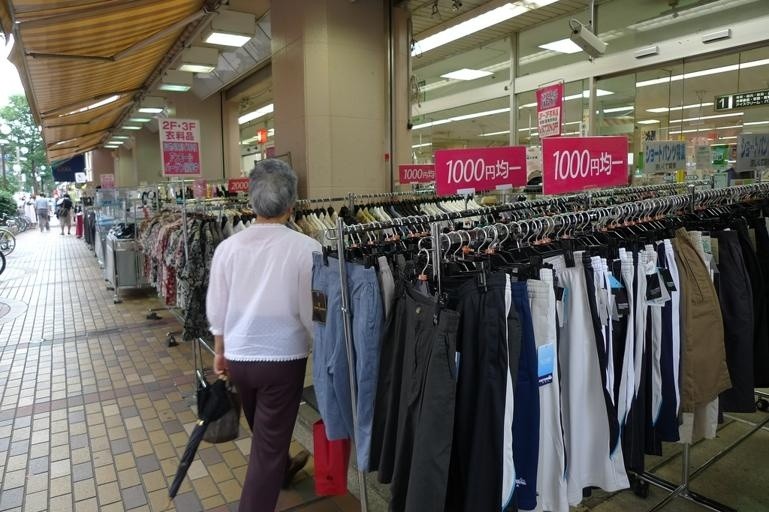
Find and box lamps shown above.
[101,8,256,148]
[450,0,462,13]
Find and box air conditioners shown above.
[702,29,729,42]
[633,46,658,59]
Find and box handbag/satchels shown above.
[203,392,240,442]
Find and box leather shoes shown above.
[282,451,308,488]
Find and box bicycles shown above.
[0,198,32,274]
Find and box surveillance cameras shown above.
[570,23,608,59]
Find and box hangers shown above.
[327,182,695,315]
[183,182,527,316]
[413,182,768,313]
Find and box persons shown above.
[20,186,100,238]
[205,160,325,512]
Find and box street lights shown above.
[40,171,46,192]
[0,139,9,191]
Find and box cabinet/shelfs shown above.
[100,227,152,305]
[92,185,157,269]
